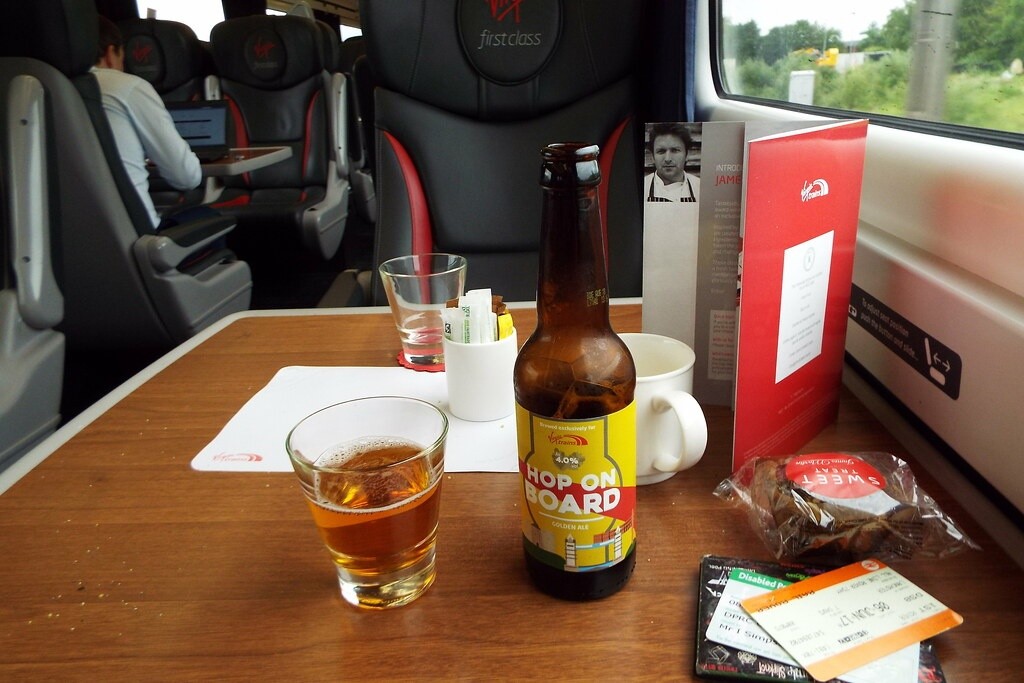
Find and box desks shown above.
[146,145,294,206]
[0,298,1024,683]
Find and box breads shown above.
[749,455,900,564]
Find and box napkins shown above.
[189,364,521,473]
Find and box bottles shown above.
[512,143,640,603]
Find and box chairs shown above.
[210,14,377,265]
[352,0,700,307]
[118,18,204,104]
[0,71,69,477]
[0,57,255,346]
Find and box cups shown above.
[286,396,449,612]
[442,327,518,421]
[617,332,708,487]
[379,253,467,367]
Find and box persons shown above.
[88,14,224,268]
[644,124,699,202]
